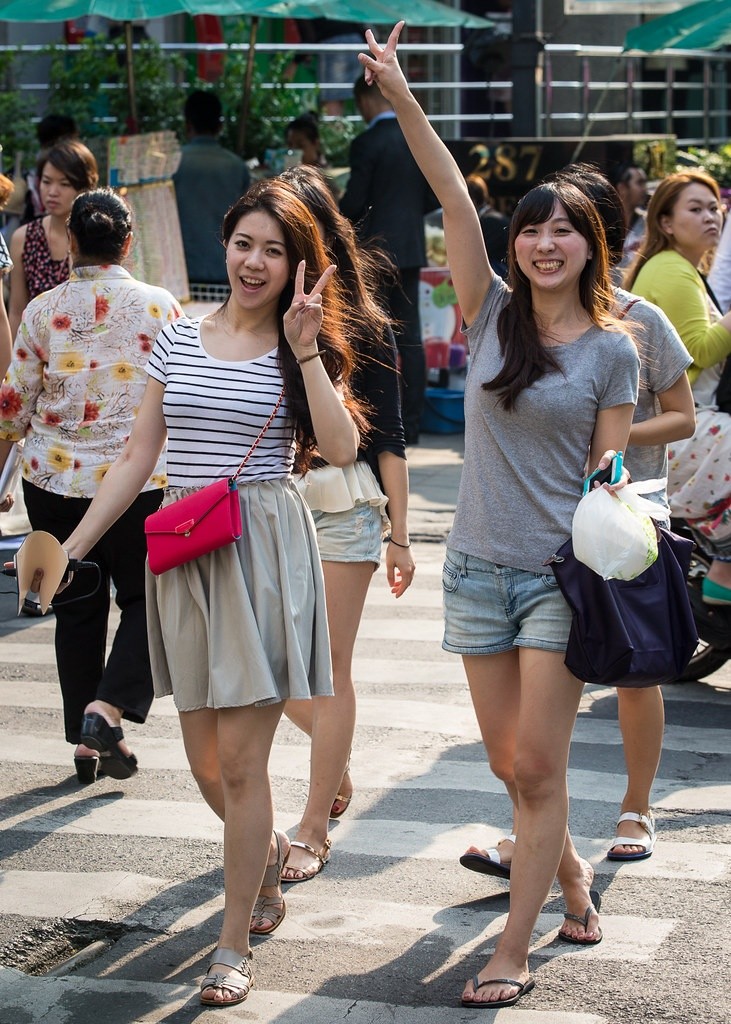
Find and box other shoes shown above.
[698,576,730,606]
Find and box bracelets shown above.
[296,350,326,364]
[389,537,411,548]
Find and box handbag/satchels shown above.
[0,442,27,508]
[143,475,245,576]
[568,475,673,583]
[544,512,701,686]
[715,351,731,415]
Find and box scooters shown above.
[662,518,731,683]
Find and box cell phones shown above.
[584,451,624,496]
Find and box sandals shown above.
[605,813,657,861]
[280,839,332,885]
[325,751,354,815]
[243,830,288,938]
[196,949,256,1007]
[459,834,517,879]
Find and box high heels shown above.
[82,710,139,780]
[74,753,108,783]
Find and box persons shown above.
[174,91,251,304]
[459,172,696,877]
[285,111,327,167]
[339,74,440,445]
[3,187,362,1006]
[272,167,416,881]
[358,21,639,1009]
[611,165,731,605]
[0,139,186,780]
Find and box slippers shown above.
[459,970,537,1008]
[556,889,604,946]
